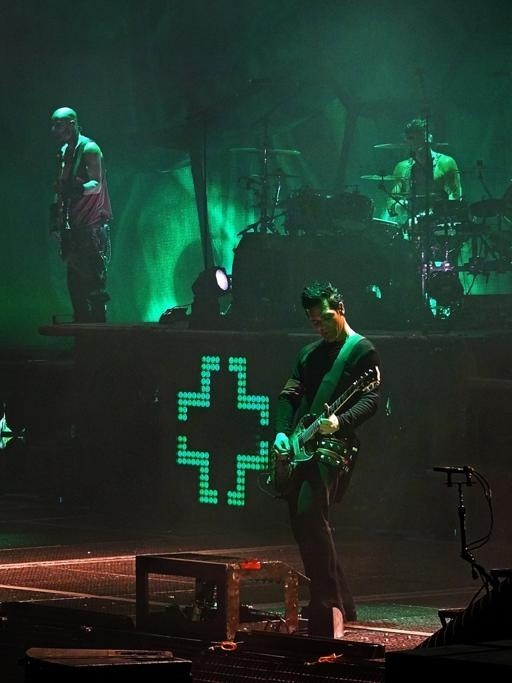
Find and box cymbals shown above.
[229,144,299,156]
[375,140,448,149]
[360,174,402,180]
[253,168,303,180]
[471,198,507,218]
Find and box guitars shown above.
[57,142,85,262]
[272,366,381,489]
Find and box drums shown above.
[332,192,375,233]
[288,187,334,236]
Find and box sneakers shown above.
[301,605,357,622]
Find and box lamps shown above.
[189,267,231,330]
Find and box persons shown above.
[268,275,382,623]
[45,103,115,324]
[376,118,463,223]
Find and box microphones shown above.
[431,465,475,474]
